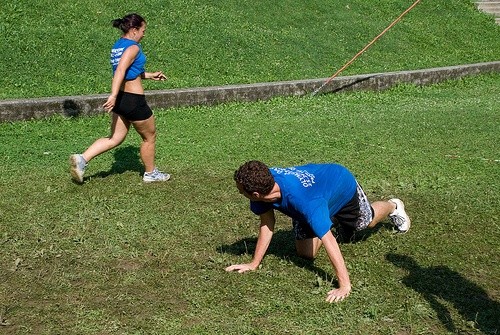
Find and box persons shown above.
[69,12,171,183]
[224,159,411,303]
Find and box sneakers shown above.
[69,154,88,184]
[143,168,170,182]
[387,198,411,232]
